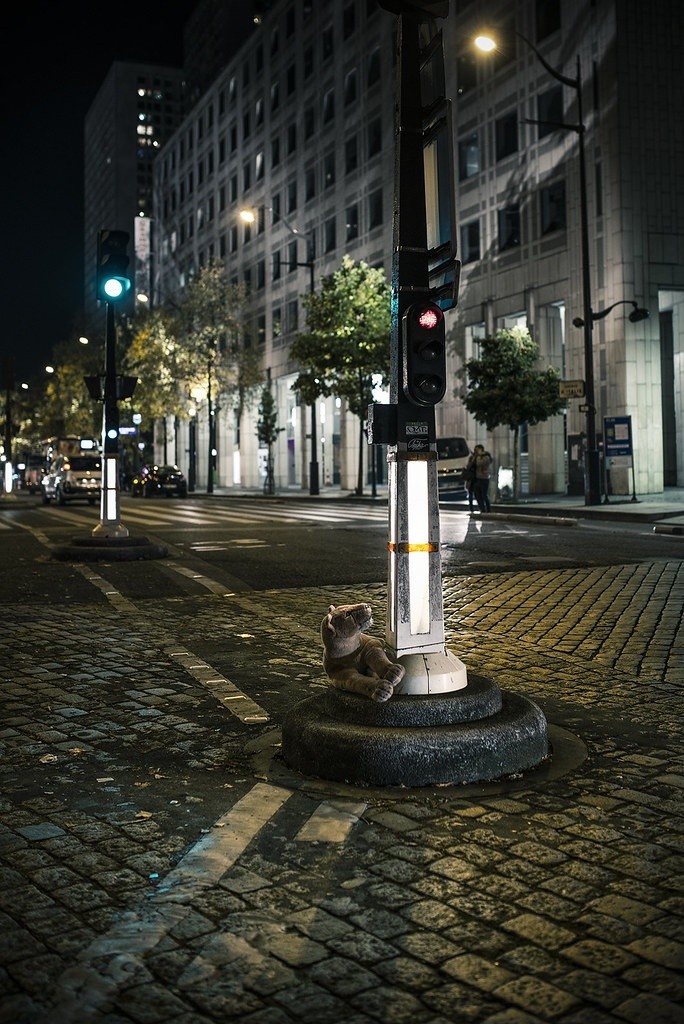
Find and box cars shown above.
[118,465,139,492]
[131,465,188,499]
[0,448,54,495]
[435,435,474,496]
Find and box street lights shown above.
[471,23,651,505]
[241,202,322,496]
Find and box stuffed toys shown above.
[319,602,406,703]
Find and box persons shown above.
[466,452,483,513]
[474,444,494,512]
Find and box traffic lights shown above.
[96,227,132,306]
[399,300,447,410]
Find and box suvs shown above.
[40,453,102,506]
